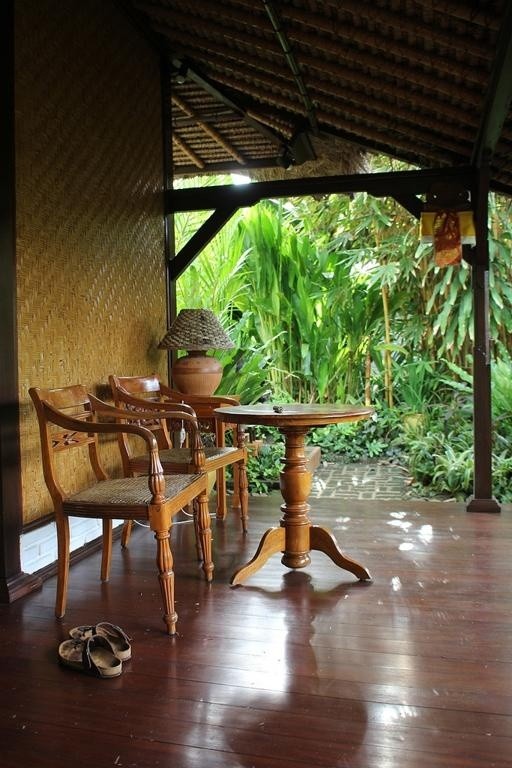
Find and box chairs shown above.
[28,374,249,636]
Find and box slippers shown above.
[59,634,122,678]
[70,622,132,662]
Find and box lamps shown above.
[156,308,236,397]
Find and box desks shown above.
[214,401,377,589]
